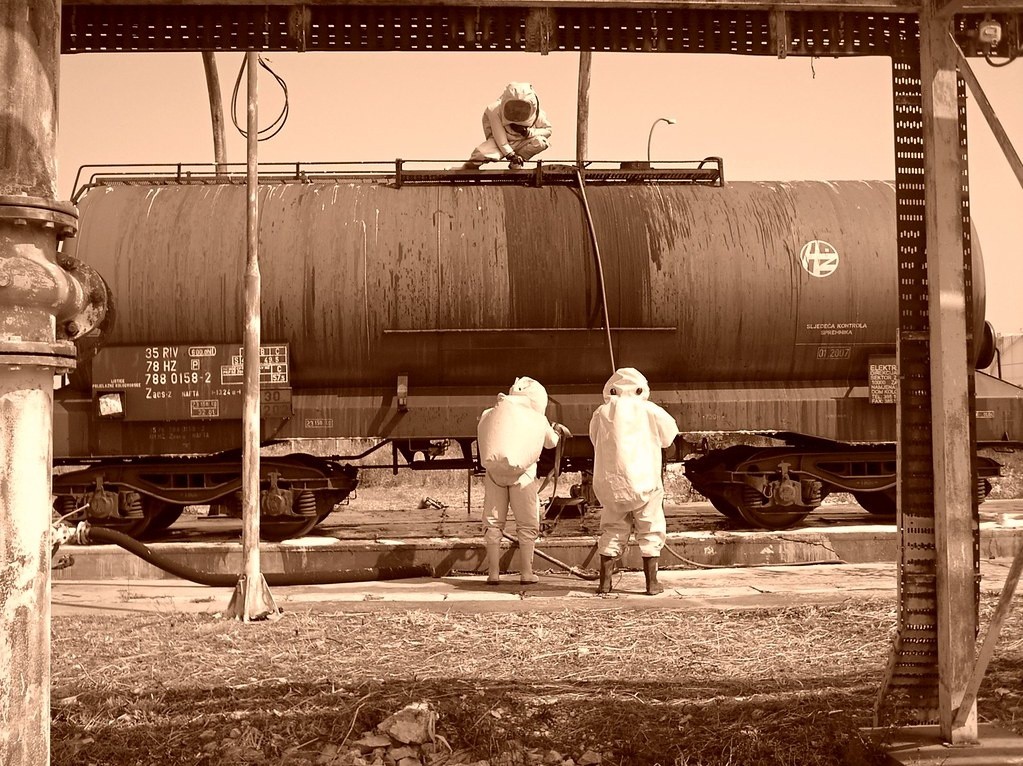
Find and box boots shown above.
[486,543,501,585]
[642,556,665,595]
[596,553,615,593]
[519,541,539,585]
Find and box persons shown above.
[480,377,562,584]
[589,367,680,596]
[462,82,552,169]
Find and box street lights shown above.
[648,117,676,183]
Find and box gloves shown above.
[506,151,526,167]
[510,123,530,138]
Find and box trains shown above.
[55,157,1023,543]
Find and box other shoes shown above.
[508,162,522,170]
[449,162,479,170]
